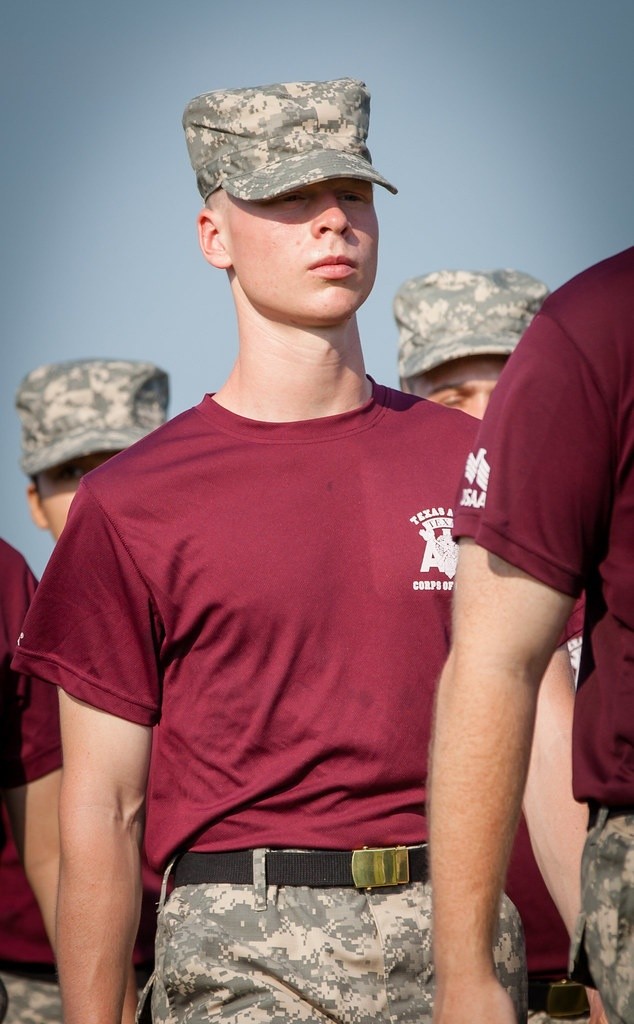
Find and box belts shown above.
[0,958,57,984]
[524,982,591,1017]
[173,844,429,887]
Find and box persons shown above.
[424,246,634,1024]
[9,78,591,1024]
[395,267,602,1024]
[0,357,172,1024]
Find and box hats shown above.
[183,79,398,202]
[15,360,169,477]
[393,268,547,377]
